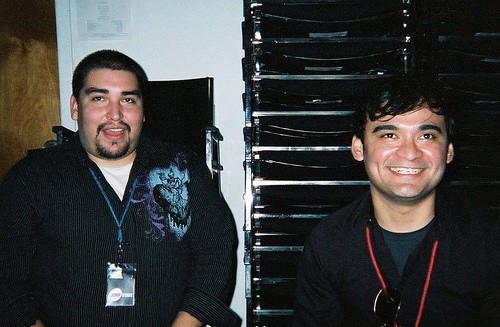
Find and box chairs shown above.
[53,0,500,327]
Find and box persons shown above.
[0,50,242,327]
[292,79,499,326]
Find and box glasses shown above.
[373,288,403,327]
